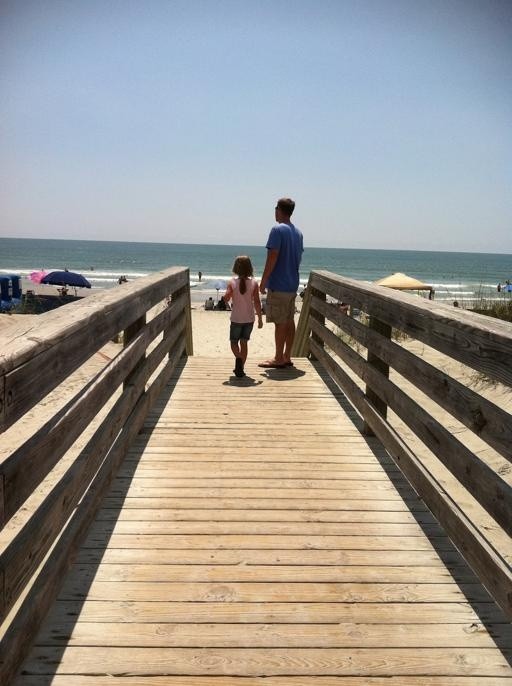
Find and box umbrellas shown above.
[30,271,49,284]
[40,271,91,297]
[204,279,227,300]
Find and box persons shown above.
[223,255,263,377]
[198,271,202,281]
[57,287,69,298]
[259,198,304,368]
[205,297,229,310]
[498,280,512,292]
[117,275,128,284]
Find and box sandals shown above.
[233,358,246,377]
[258,358,293,369]
